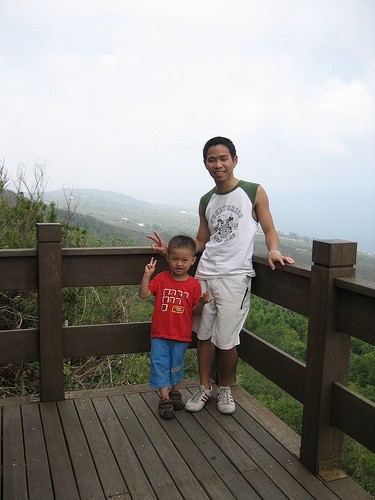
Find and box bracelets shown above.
[269,251,281,256]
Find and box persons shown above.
[140,235,215,420]
[146,137,294,413]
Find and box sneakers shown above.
[185,382,212,412]
[216,385,235,414]
[169,390,185,410]
[158,398,174,419]
